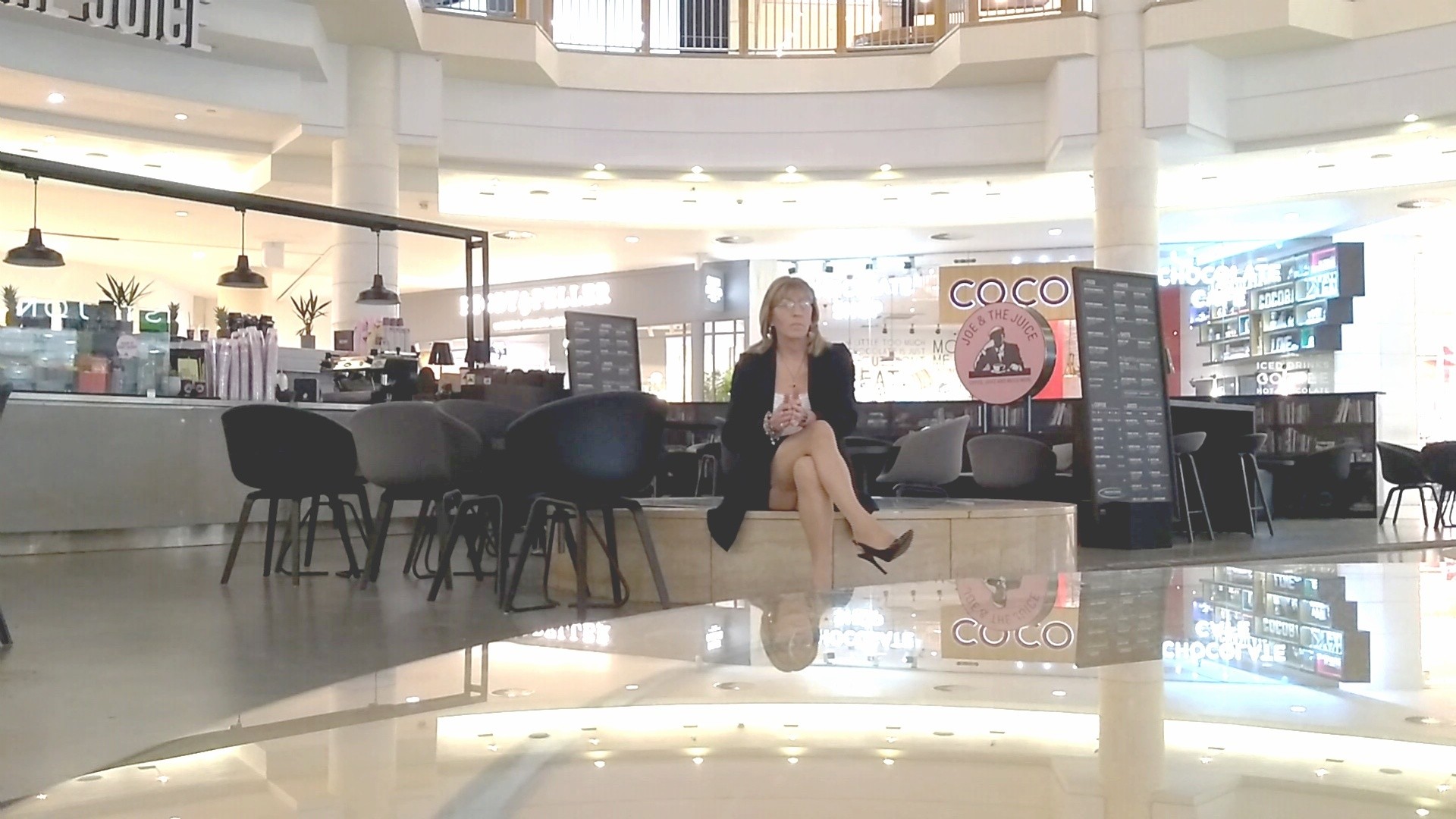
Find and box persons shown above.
[705,276,914,592]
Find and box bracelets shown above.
[763,410,780,447]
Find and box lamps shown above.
[351,229,403,306]
[1,173,67,268]
[214,205,268,290]
[426,343,454,399]
[463,342,491,375]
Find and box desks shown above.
[1256,457,1295,472]
[838,435,889,498]
[661,417,718,454]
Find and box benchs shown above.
[653,402,984,497]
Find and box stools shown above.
[1227,433,1275,540]
[1171,428,1216,545]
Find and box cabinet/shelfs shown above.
[1287,391,1383,522]
[1217,394,1294,521]
[987,399,1078,496]
[1188,240,1364,363]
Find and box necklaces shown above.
[776,345,807,387]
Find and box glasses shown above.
[770,297,813,310]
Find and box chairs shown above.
[346,401,512,602]
[1294,441,1350,519]
[219,403,380,590]
[963,432,1057,500]
[493,389,674,611]
[871,416,972,498]
[1421,437,1456,530]
[400,396,551,582]
[1377,440,1447,529]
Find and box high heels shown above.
[851,529,914,575]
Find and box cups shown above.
[162,377,182,398]
[1257,290,1293,309]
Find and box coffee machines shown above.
[317,344,420,404]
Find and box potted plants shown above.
[169,303,189,342]
[95,270,153,324]
[1,285,23,327]
[289,289,332,350]
[213,306,231,339]
